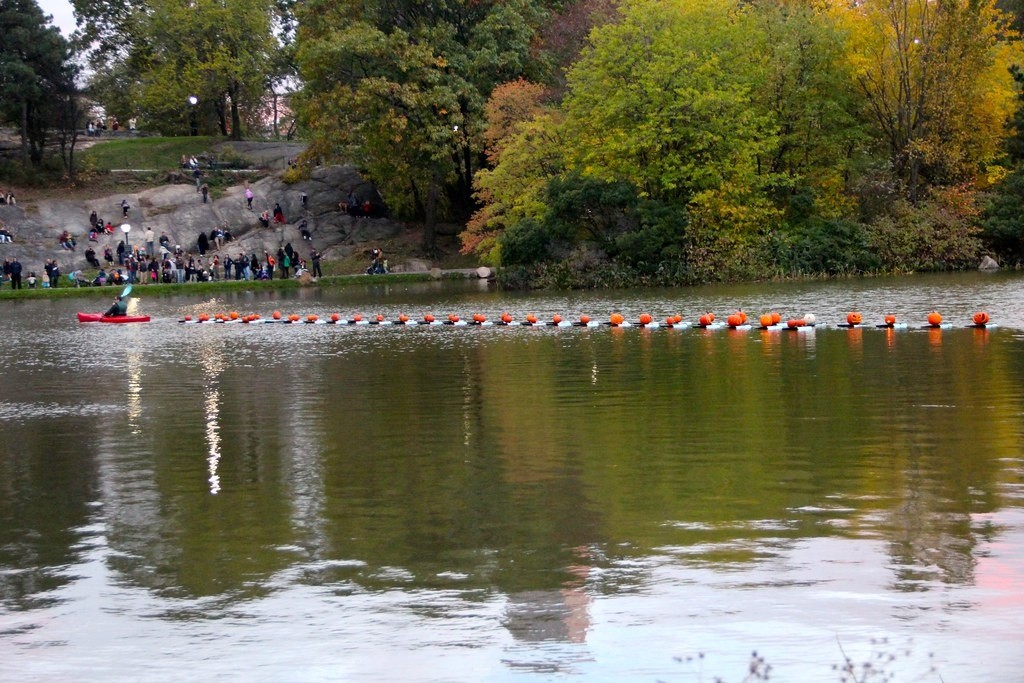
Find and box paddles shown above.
[97,285,133,322]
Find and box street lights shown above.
[190,96,198,135]
[121,224,131,245]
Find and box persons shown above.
[0,114,389,289]
[102,296,127,316]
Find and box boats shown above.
[77,312,150,323]
